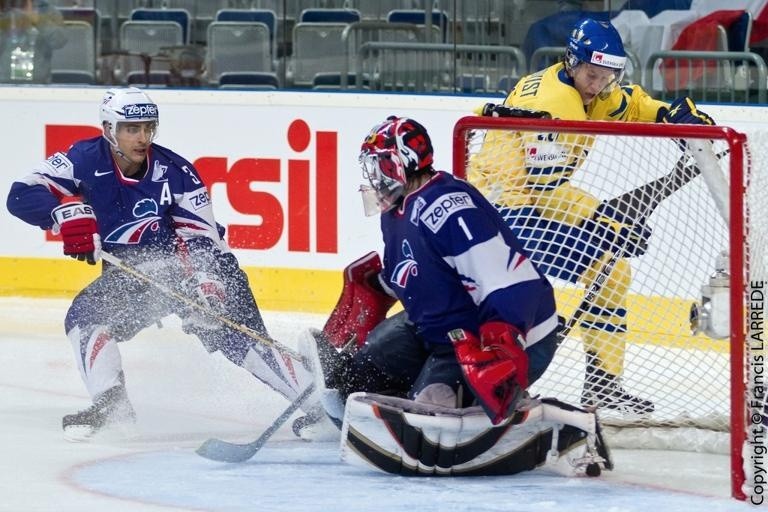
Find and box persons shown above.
[459,21,719,414]
[7,87,319,445]
[521,0,767,93]
[299,113,614,481]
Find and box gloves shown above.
[51,200,102,265]
[185,271,227,331]
[594,218,653,254]
[664,94,716,150]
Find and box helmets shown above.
[101,87,158,146]
[359,116,433,216]
[567,18,627,70]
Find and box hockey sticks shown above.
[196,332,358,462]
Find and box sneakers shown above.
[582,381,653,413]
[63,386,137,431]
